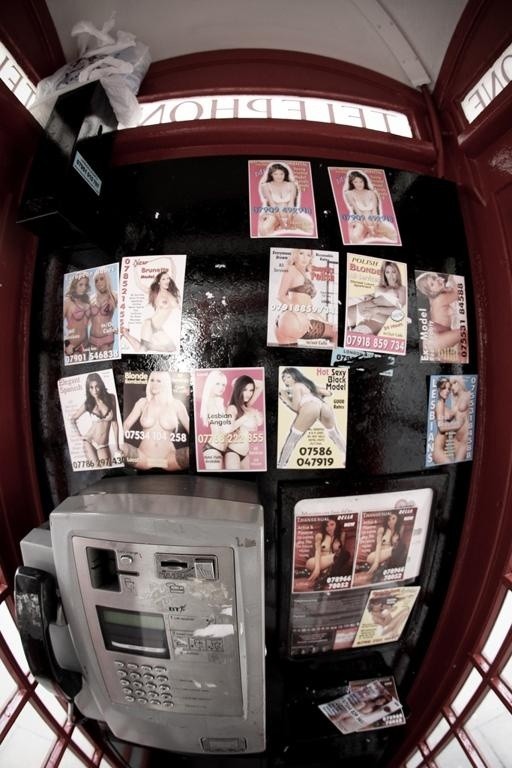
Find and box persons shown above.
[62,164,472,734]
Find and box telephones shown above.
[11,470,270,759]
